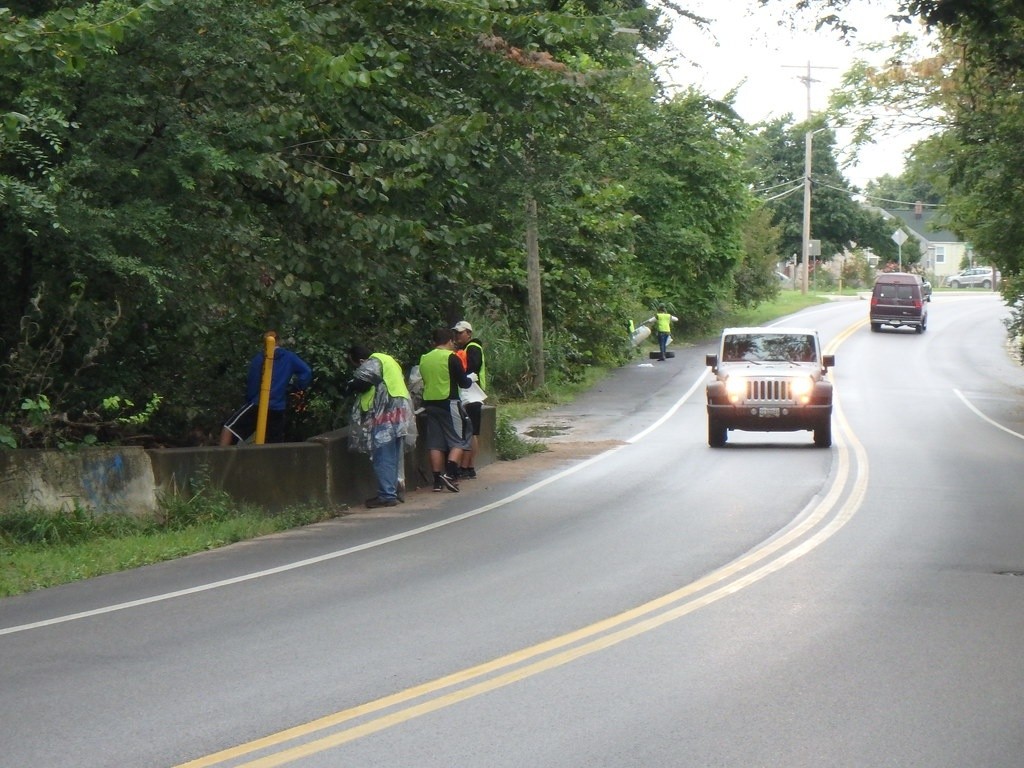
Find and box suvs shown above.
[945,267,1001,289]
[706,326,835,448]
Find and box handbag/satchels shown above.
[665,336,673,346]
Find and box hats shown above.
[451,321,472,332]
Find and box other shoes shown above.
[457,468,477,479]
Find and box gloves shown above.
[467,372,479,383]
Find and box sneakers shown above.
[433,485,442,491]
[438,472,460,491]
[366,497,397,508]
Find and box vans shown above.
[869,272,927,334]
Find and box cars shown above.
[914,274,931,303]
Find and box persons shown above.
[215,332,313,450]
[419,328,480,492]
[450,321,487,479]
[641,303,679,362]
[625,319,635,337]
[346,345,413,510]
